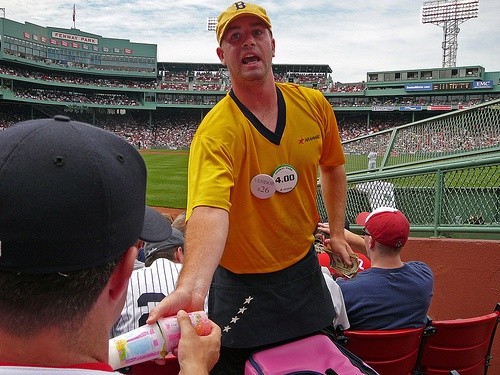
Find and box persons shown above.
[314,206,433,330]
[367,147,377,173]
[108,211,208,375]
[354,179,396,211]
[146,1,355,375]
[0,115,221,375]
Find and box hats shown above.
[0,115,172,264]
[356,207,410,247]
[215,1,272,47]
[144,228,184,259]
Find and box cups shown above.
[108,322,167,370]
[157,310,212,353]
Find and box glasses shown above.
[362,228,370,236]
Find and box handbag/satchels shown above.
[244,334,380,375]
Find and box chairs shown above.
[119,355,182,375]
[336,302,500,375]
